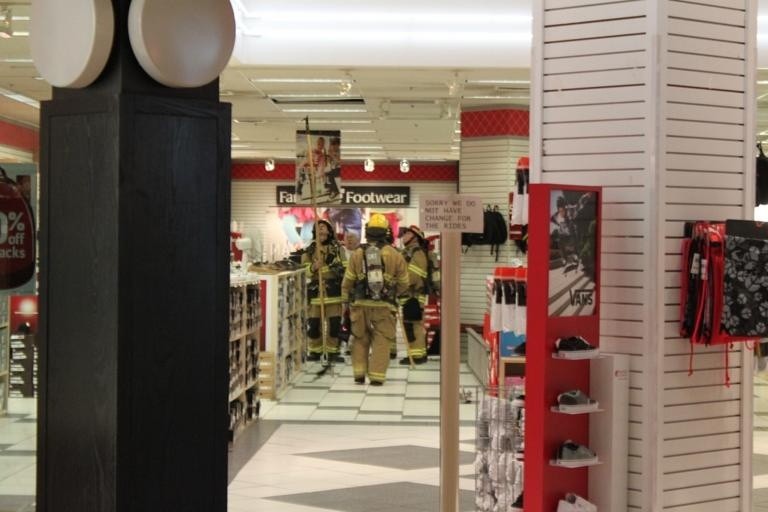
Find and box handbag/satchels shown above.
[462,205,507,262]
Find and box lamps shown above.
[264,159,275,171]
[364,157,374,172]
[399,157,410,172]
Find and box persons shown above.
[549,192,595,268]
[296,137,341,203]
[300,213,433,387]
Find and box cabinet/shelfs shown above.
[265,267,310,395]
[552,349,609,512]
[230,279,260,443]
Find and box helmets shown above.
[406,225,426,241]
[367,215,389,236]
[313,218,335,241]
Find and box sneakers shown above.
[556,439,598,466]
[558,336,599,359]
[557,493,597,512]
[558,389,599,413]
